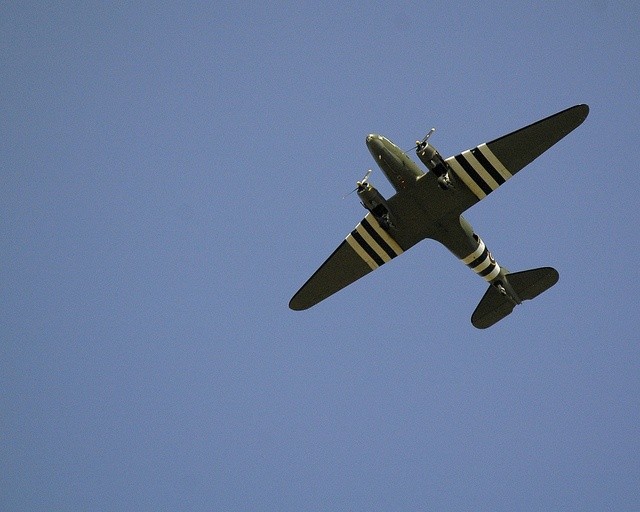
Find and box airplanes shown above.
[288,103,590,329]
